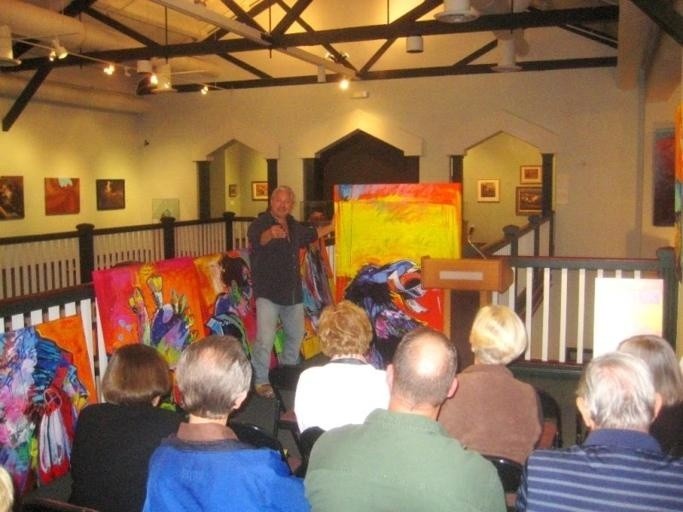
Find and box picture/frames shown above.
[476,177,501,203]
[95,178,126,211]
[514,186,545,216]
[229,184,238,198]
[250,180,271,202]
[519,164,544,184]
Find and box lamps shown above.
[0,26,230,97]
[404,31,424,57]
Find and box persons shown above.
[69,344,189,511]
[142,336,308,511]
[302,329,507,512]
[512,353,683,511]
[293,301,390,433]
[614,335,681,461]
[246,186,335,398]
[435,304,544,511]
[1,179,24,220]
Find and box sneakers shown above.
[255,383,275,400]
